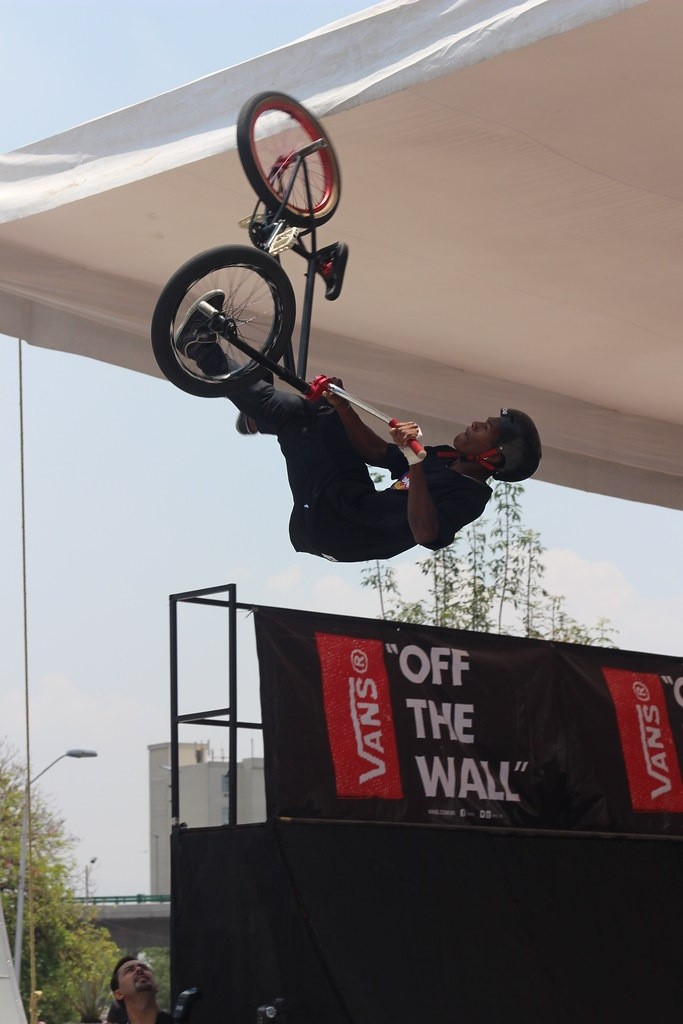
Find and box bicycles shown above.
[148,84,431,467]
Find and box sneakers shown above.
[175,289,225,359]
[235,370,273,434]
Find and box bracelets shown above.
[336,403,351,411]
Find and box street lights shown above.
[12,746,100,987]
[85,856,98,907]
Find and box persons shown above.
[111,955,171,1024]
[174,290,540,562]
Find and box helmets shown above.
[492,407,542,482]
